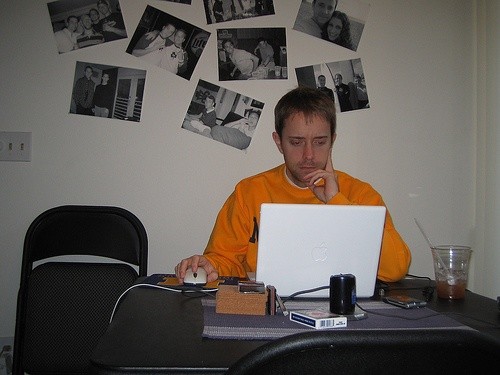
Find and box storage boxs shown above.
[288,309,348,330]
[214,285,269,318]
[239,280,266,294]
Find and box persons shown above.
[73,66,95,115]
[55,0,127,53]
[296,0,337,37]
[208,0,255,23]
[92,73,114,117]
[132,22,188,74]
[225,109,261,137]
[322,11,353,50]
[189,95,216,126]
[335,73,352,112]
[175,88,411,282]
[222,39,260,80]
[318,75,334,103]
[354,75,369,108]
[253,37,275,68]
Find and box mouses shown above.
[182,265,209,286]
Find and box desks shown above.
[89,275,500,375]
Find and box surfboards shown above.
[320,63,341,113]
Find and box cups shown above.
[430,245,472,300]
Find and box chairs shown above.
[11,204,148,375]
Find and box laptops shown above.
[247,202,386,299]
